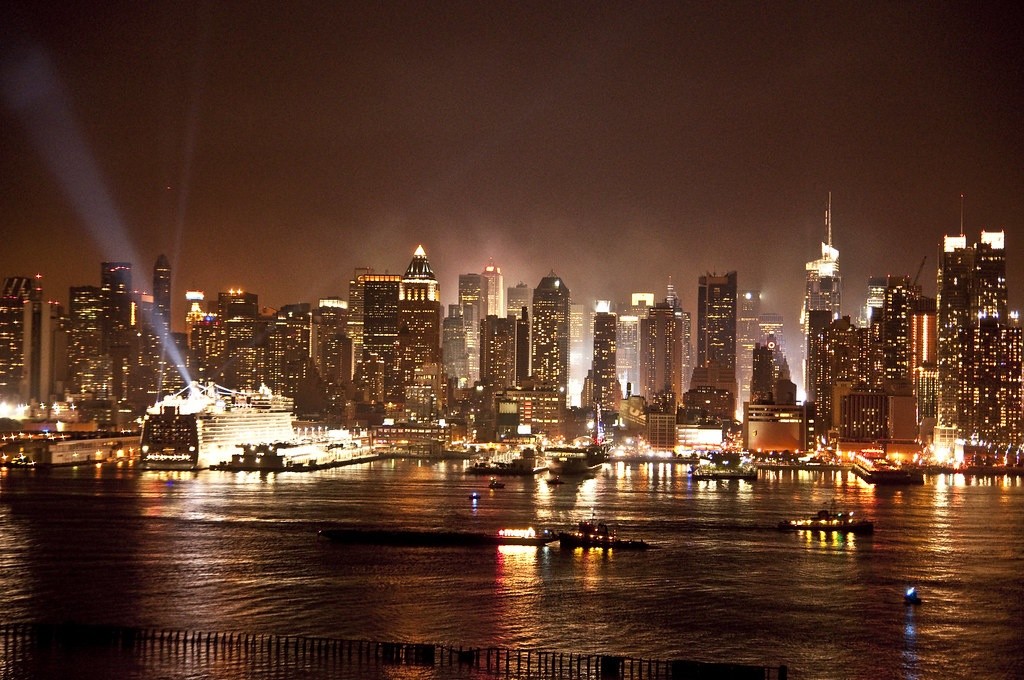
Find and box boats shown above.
[469,494,481,499]
[135,379,296,470]
[688,462,758,480]
[548,454,603,485]
[556,518,650,550]
[465,446,550,476]
[545,475,565,486]
[488,477,505,488]
[779,509,877,532]
